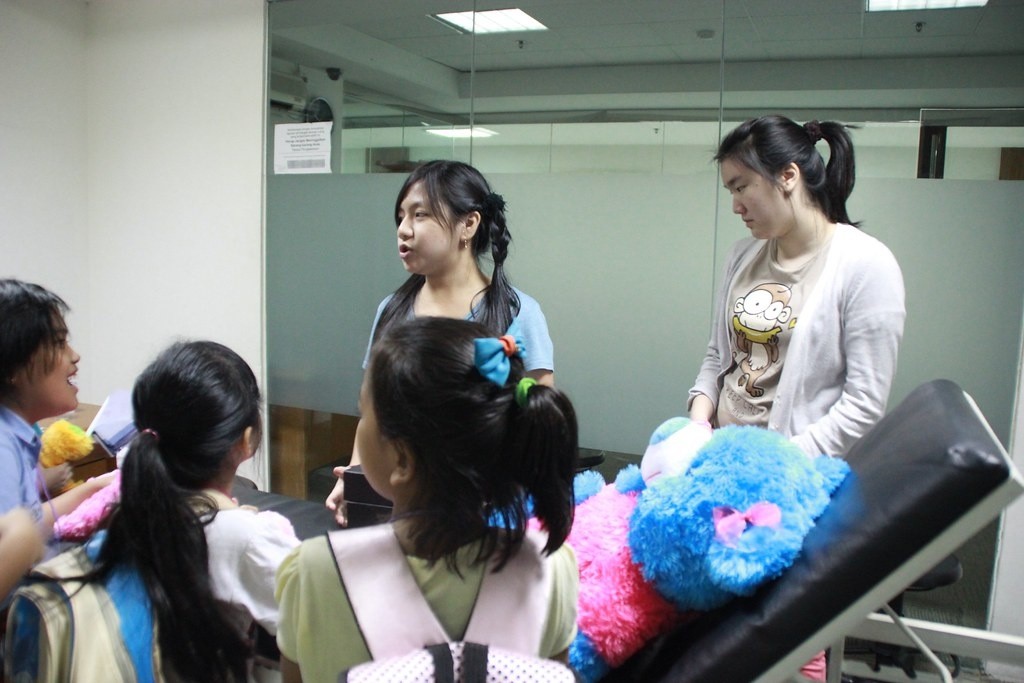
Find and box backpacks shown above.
[345,637,579,683]
[4,529,163,683]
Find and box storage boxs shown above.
[344,465,393,528]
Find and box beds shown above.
[186,381,1024,682]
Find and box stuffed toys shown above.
[39,419,95,501]
[486,416,851,683]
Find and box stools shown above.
[826,554,962,679]
[577,448,606,474]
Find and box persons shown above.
[273,316,579,683]
[1,278,121,682]
[687,116,906,682]
[325,159,556,528]
[52,342,303,683]
